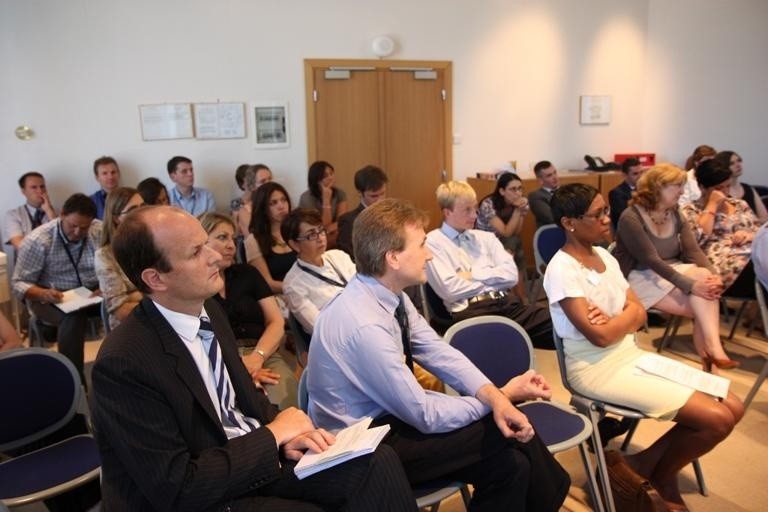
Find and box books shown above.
[293,423,391,480]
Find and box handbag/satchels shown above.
[596,450,672,512]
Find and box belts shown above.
[468,290,507,303]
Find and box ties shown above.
[199,317,256,433]
[396,300,414,375]
[32,211,42,230]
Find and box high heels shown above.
[704,347,740,371]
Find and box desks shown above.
[468,167,652,282]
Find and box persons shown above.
[0,158,170,392]
[609,148,768,375]
[167,156,389,395]
[306,198,572,512]
[542,184,745,512]
[91,205,418,512]
[529,161,556,226]
[476,171,530,306]
[426,181,557,351]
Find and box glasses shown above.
[580,206,610,219]
[295,228,327,241]
